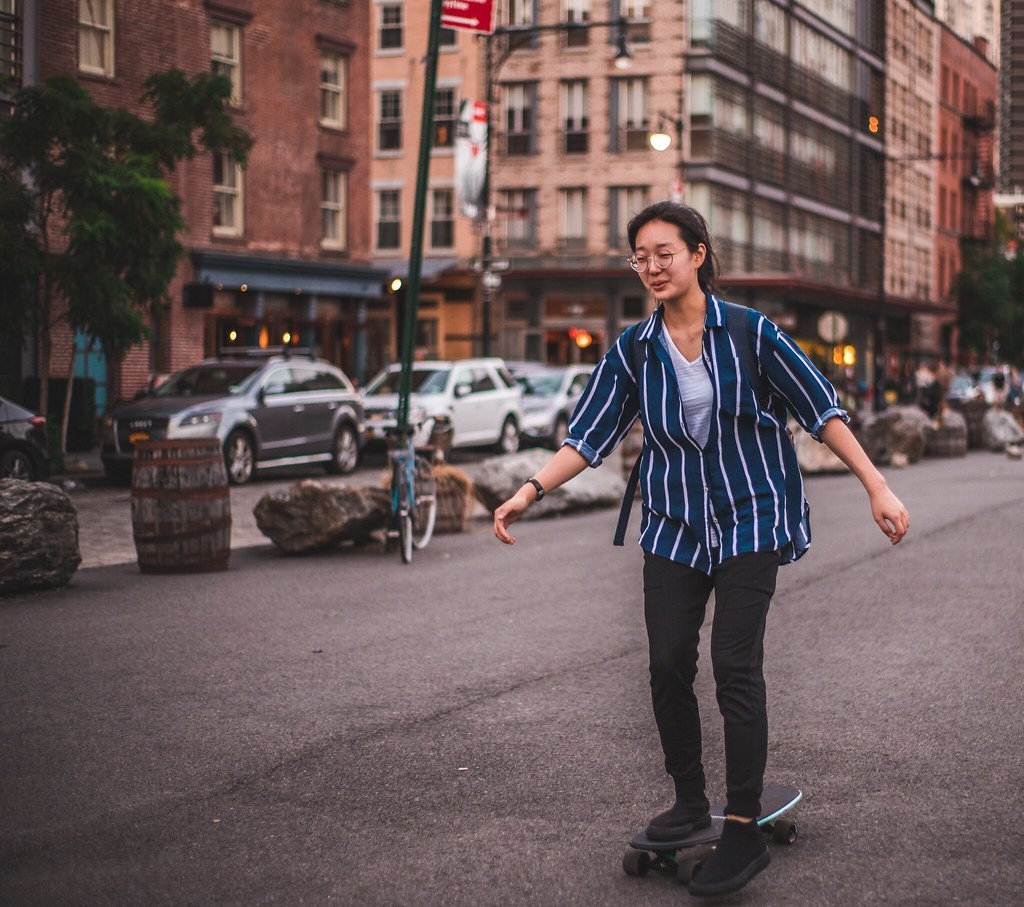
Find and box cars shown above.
[360,355,524,466]
[504,358,604,451]
[0,396,54,483]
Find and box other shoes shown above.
[688,818,772,898]
[646,788,713,842]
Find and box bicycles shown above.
[379,415,440,564]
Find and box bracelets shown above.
[524,477,545,502]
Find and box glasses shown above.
[627,246,690,273]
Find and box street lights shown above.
[853,147,989,404]
[472,12,654,358]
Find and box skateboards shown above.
[622,784,804,885]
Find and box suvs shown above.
[96,343,368,488]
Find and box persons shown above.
[492,201,912,896]
[879,354,1024,457]
